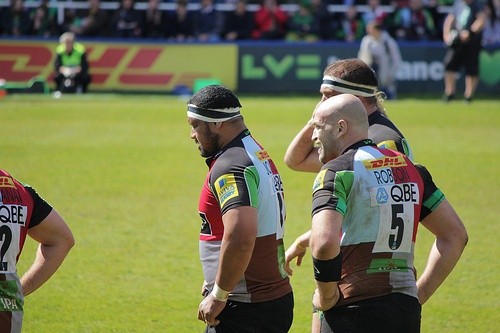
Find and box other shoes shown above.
[441,95,472,103]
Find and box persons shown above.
[1,162,75,333]
[186,83,295,333]
[284,57,410,277]
[1,1,500,48]
[441,1,488,104]
[356,21,405,100]
[309,93,469,333]
[52,33,93,99]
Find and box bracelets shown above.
[295,239,307,252]
